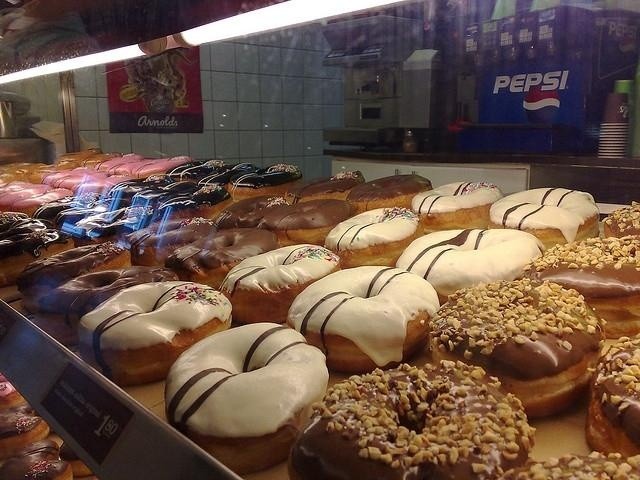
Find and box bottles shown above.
[402,125,419,154]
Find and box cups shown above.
[597,79,637,159]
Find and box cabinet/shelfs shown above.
[0,0,640,480]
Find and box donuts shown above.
[0,148,640,480]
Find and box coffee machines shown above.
[321,40,458,138]
